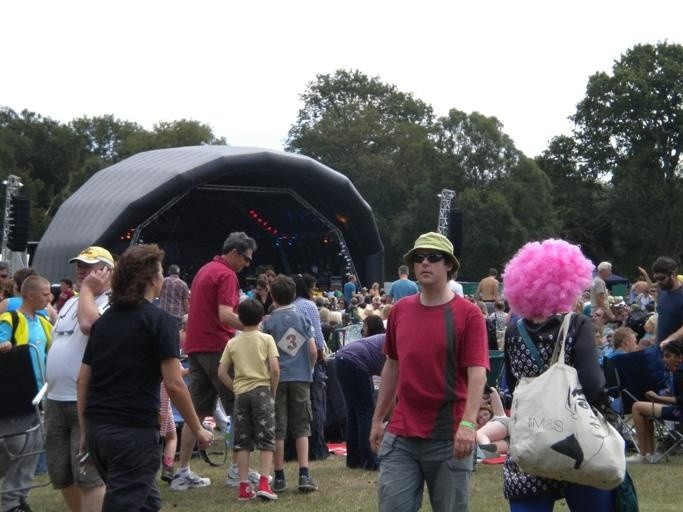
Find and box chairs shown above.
[0,343,59,497]
[602,346,683,467]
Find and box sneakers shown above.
[625,454,646,465]
[160,468,317,501]
[647,452,662,464]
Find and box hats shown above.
[402,231,460,277]
[69,245,115,270]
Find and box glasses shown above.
[651,290,656,292]
[0,274,7,278]
[54,315,78,336]
[235,252,253,262]
[410,252,445,263]
[652,273,666,283]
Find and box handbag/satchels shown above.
[507,362,626,491]
[611,470,638,512]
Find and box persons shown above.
[369,231,491,512]
[334,259,682,470]
[0,227,357,512]
[501,238,625,512]
